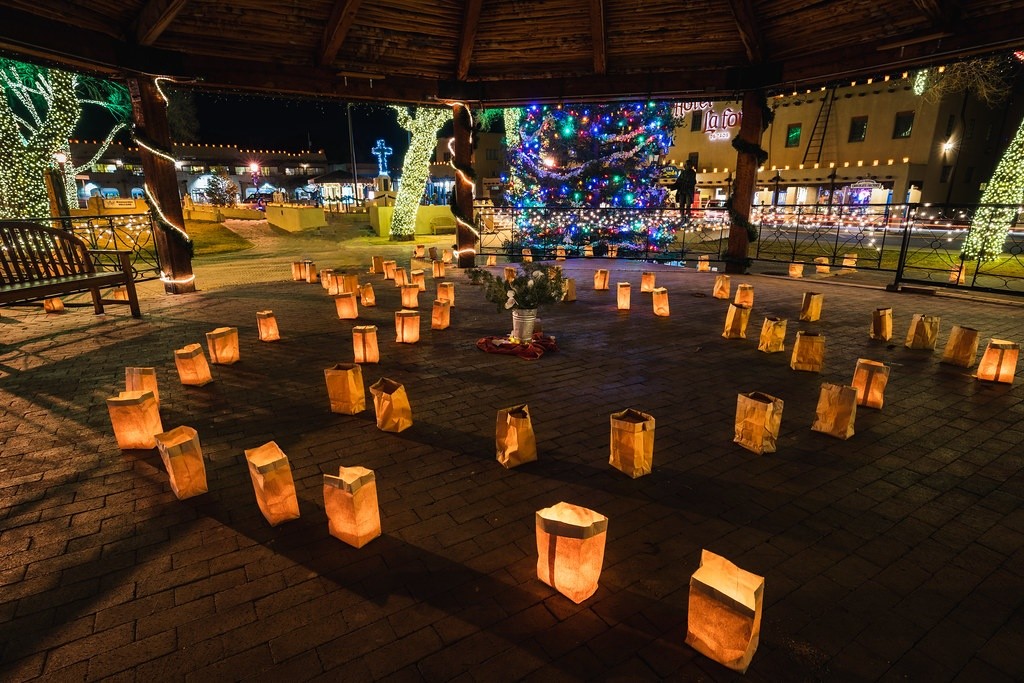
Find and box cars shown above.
[242,192,272,203]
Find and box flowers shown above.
[465,260,569,313]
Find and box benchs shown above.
[0,219,142,318]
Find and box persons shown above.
[668,160,697,216]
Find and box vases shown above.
[511,308,538,343]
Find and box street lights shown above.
[249,162,260,206]
